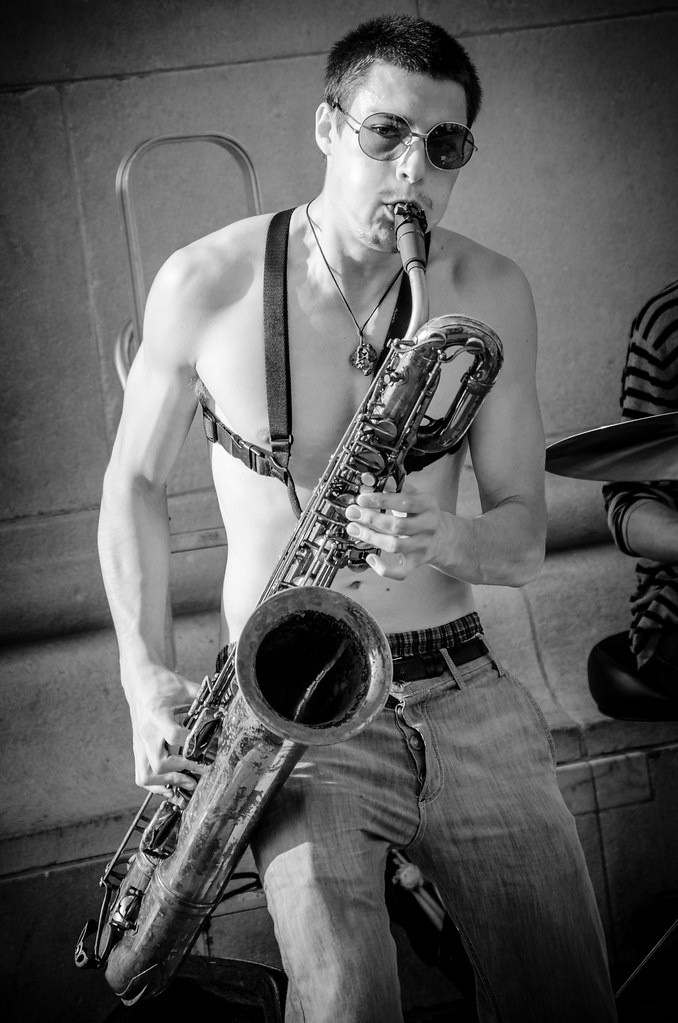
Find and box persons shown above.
[96,13,616,1023]
[586,279,678,719]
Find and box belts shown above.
[392,636,488,682]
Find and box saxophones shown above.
[74,198,508,1008]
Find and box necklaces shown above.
[307,198,404,376]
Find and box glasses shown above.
[328,94,478,171]
